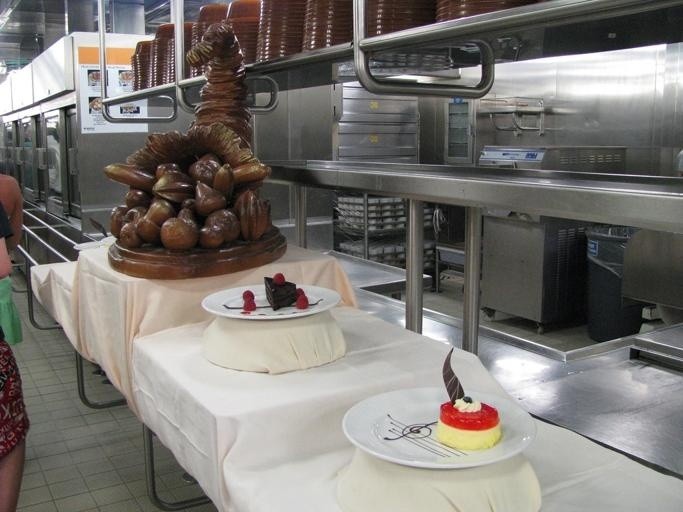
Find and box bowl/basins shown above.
[337,195,435,266]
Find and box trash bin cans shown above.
[586,226,643,343]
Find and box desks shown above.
[76,234,682,510]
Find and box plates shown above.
[202,284,340,319]
[342,387,535,469]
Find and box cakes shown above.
[438,347,501,450]
[263,274,297,311]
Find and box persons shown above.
[0,173,24,255]
[0,204,35,511]
